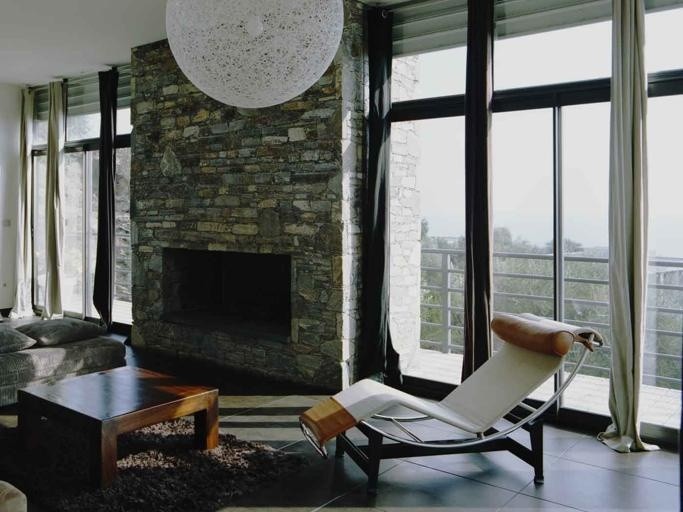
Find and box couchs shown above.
[0,306,131,406]
[0,480,28,512]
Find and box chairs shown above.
[297,310,606,503]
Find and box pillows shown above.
[0,323,37,353]
[15,316,106,346]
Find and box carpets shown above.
[1,413,307,512]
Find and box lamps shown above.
[163,0,342,111]
